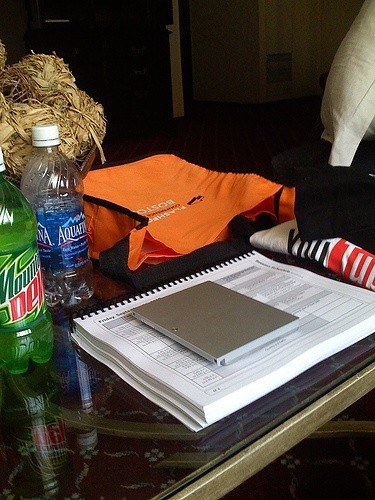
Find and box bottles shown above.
[0,148,55,375]
[20,122,95,308]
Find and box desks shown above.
[0,255,375,500]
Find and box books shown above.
[133,281,301,370]
